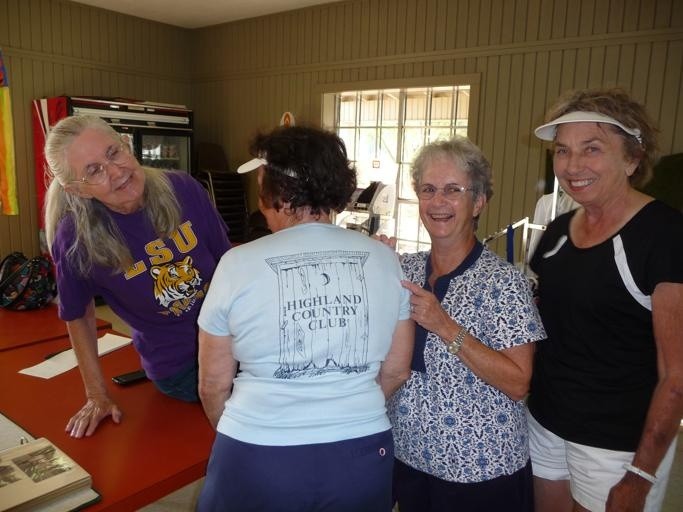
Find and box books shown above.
[0,411,103,512]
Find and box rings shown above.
[411,304,416,313]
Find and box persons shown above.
[193,124,418,509]
[39,111,234,443]
[511,89,683,511]
[362,134,549,510]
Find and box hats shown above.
[534,111,642,144]
[237,151,317,186]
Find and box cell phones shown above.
[111,369,147,385]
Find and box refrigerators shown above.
[30,94,199,305]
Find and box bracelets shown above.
[445,329,468,355]
[622,462,657,486]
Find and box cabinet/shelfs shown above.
[32,94,194,283]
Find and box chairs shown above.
[191,144,248,243]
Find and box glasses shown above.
[71,142,126,185]
[416,181,475,200]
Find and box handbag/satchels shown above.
[0,252,57,311]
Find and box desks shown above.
[0,329,215,511]
[1,306,113,352]
